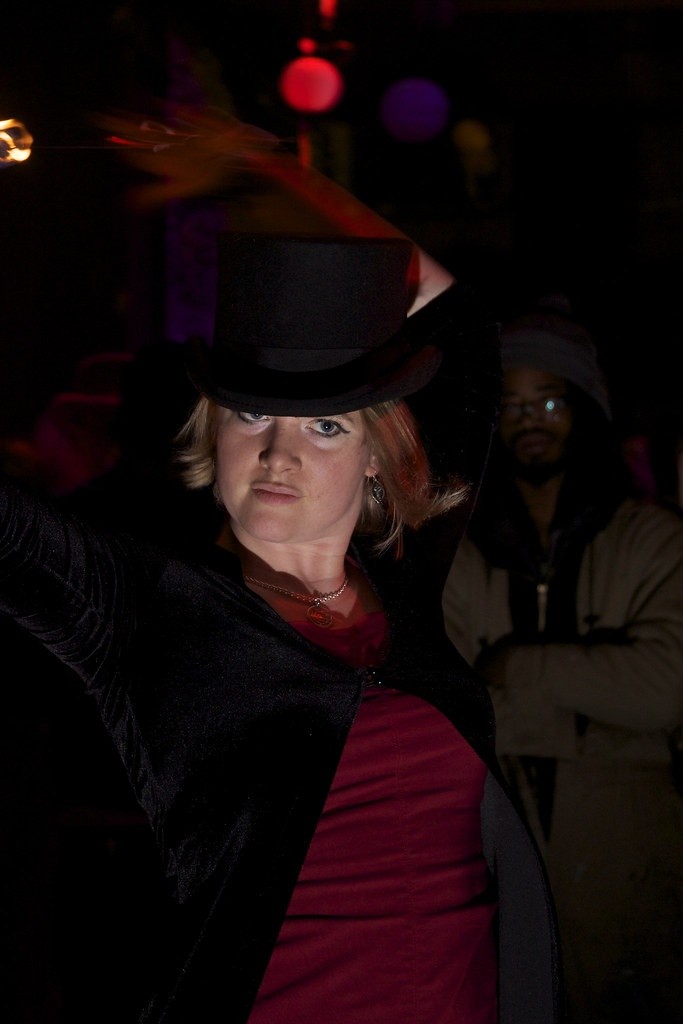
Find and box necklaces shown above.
[243,569,350,628]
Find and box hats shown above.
[182,230,443,418]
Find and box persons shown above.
[0,235,562,1024]
[443,296,683,1024]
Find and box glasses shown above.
[496,396,566,424]
